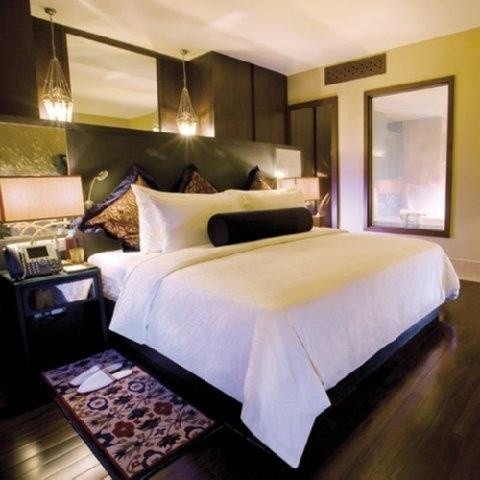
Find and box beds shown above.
[65,123,460,470]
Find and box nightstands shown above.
[1,259,110,369]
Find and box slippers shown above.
[77,368,132,396]
[69,363,123,388]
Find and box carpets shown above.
[40,349,225,479]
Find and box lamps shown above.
[0,174,86,252]
[176,46,197,137]
[41,8,74,121]
[277,176,321,200]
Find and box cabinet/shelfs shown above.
[194,50,288,143]
[0,0,38,116]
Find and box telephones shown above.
[3,240,63,281]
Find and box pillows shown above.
[78,165,313,254]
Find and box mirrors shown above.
[65,30,159,132]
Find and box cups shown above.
[66,236,84,264]
